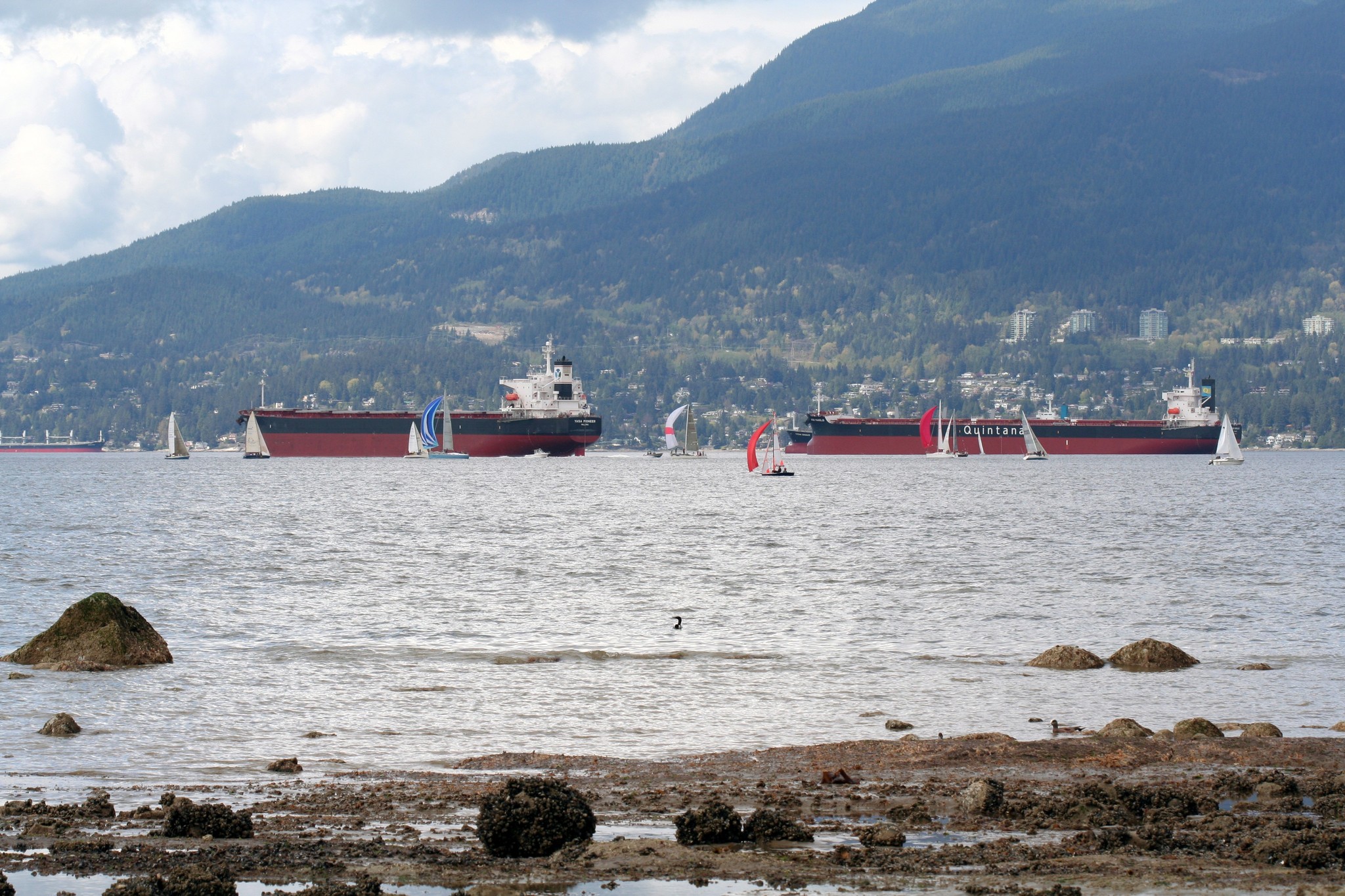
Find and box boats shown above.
[785,357,1243,455]
[235,333,602,458]
[525,448,551,457]
[0,429,106,453]
[643,451,663,457]
[505,393,520,401]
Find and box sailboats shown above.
[243,411,270,459]
[1208,411,1245,466]
[919,399,968,458]
[747,409,795,476]
[1021,410,1049,461]
[165,411,190,460]
[404,390,470,459]
[665,404,708,459]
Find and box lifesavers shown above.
[1214,421,1222,427]
